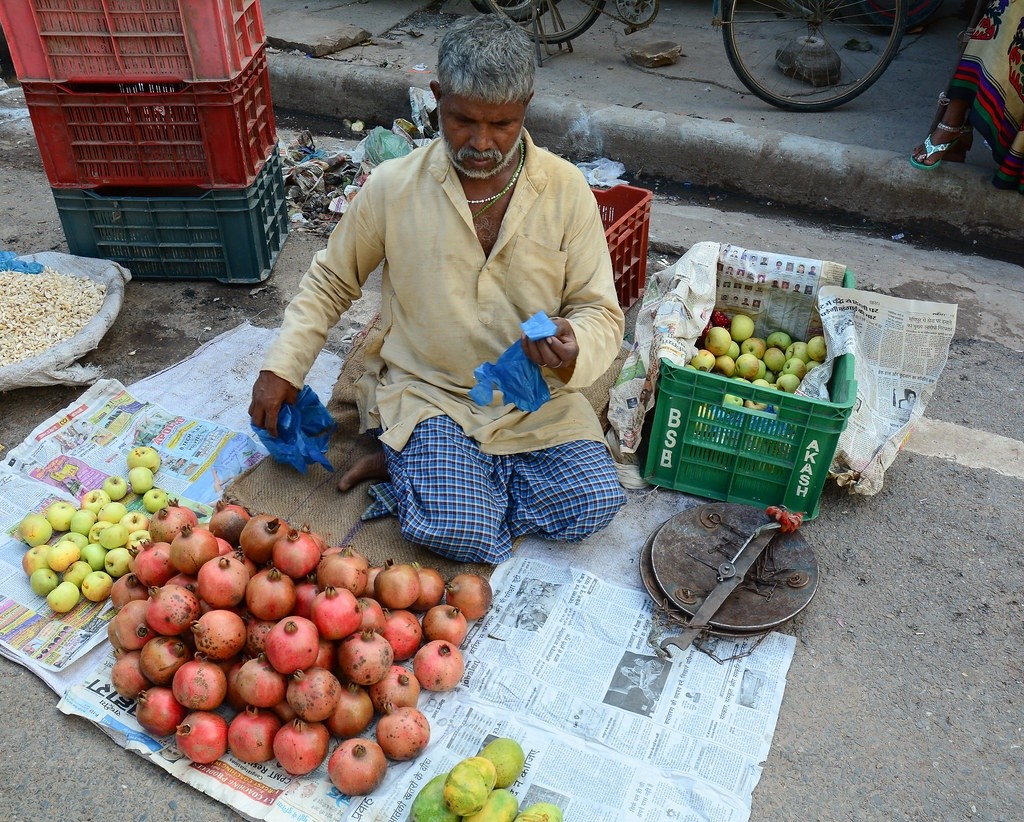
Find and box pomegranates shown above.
[110,499,492,796]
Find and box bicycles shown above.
[486,0,906,113]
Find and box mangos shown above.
[412,737,562,821]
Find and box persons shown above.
[249,12,625,563]
[910,0,1024,193]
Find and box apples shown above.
[21,447,168,612]
[686,314,826,412]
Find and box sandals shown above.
[910,122,971,170]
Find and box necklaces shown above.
[466,141,524,219]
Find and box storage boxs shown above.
[641,243,856,522]
[592,184,653,315]
[0,0,291,284]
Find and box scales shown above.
[640,502,820,664]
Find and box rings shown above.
[537,362,551,368]
[550,359,563,370]
